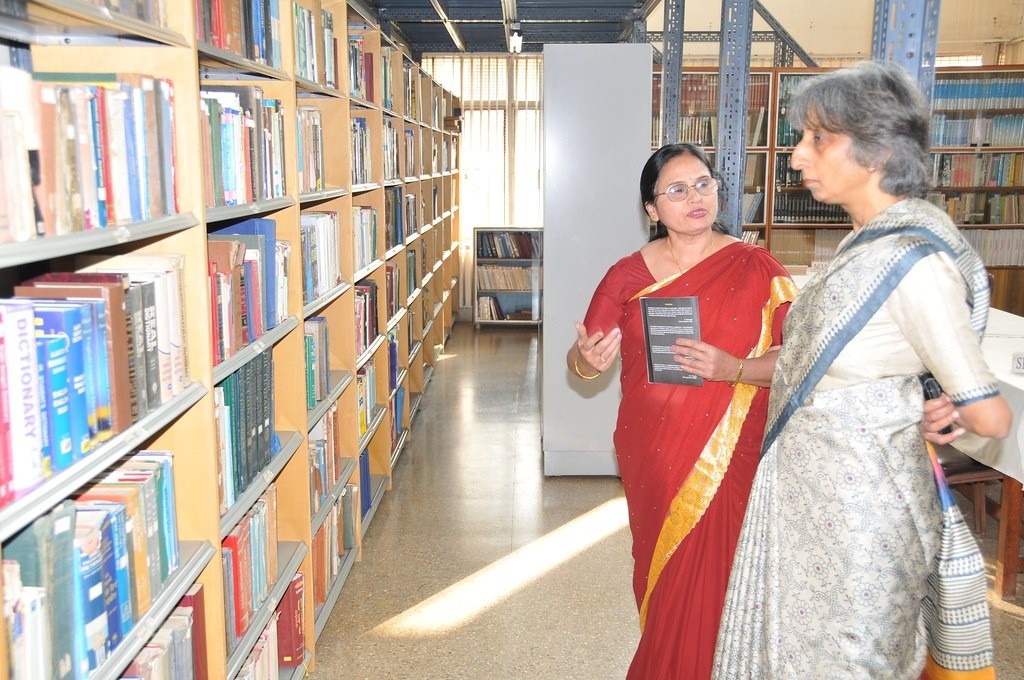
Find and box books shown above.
[775,74,1024,224]
[510,310,532,320]
[771,229,1024,266]
[652,73,768,246]
[0,2,464,680]
[478,296,505,320]
[640,296,704,387]
[478,231,533,259]
[478,264,542,290]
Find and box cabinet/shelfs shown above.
[0,0,464,680]
[651,66,1024,271]
[472,226,543,333]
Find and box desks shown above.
[947,306,1024,603]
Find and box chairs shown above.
[931,442,1004,534]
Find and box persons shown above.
[708,62,1010,680]
[568,144,796,680]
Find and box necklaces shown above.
[666,237,714,271]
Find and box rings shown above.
[600,356,606,362]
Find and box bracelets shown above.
[727,358,743,387]
[574,361,601,380]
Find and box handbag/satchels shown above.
[919,440,996,680]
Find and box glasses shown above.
[653,178,720,201]
